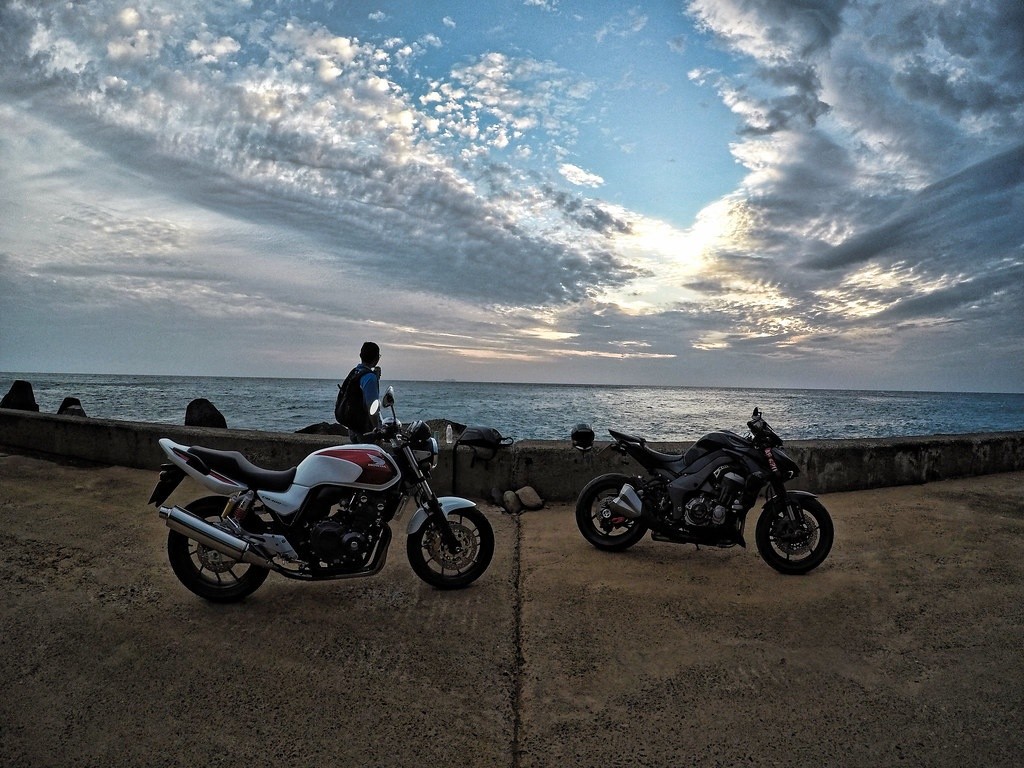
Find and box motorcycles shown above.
[147,385,496,601]
[573,407,835,575]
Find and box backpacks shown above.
[335,368,374,431]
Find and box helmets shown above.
[571,424,594,451]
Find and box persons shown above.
[335,342,381,443]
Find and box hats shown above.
[361,342,379,355]
